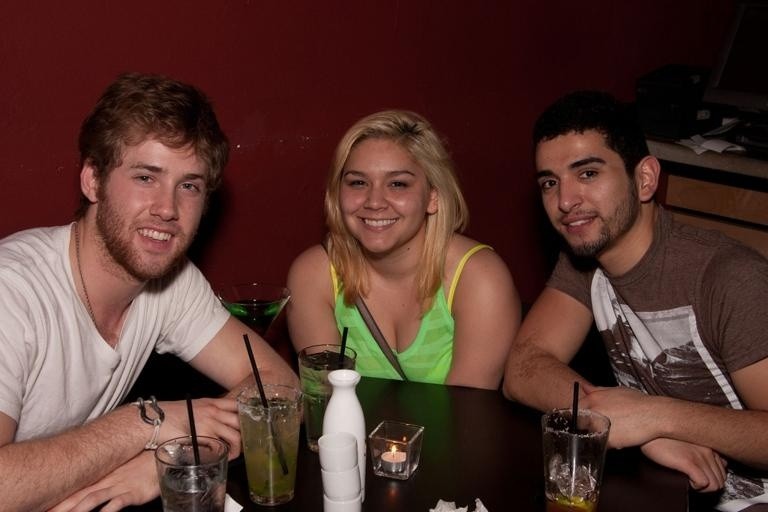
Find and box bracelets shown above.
[134,396,167,449]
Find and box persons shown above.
[502,93,768,493]
[286,110,520,390]
[1,73,303,511]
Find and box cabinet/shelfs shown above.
[644,96,768,263]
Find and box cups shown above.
[218,285,291,335]
[298,344,356,451]
[236,384,303,506]
[369,420,425,479]
[321,465,360,500]
[318,432,358,472]
[323,494,362,512]
[542,408,611,512]
[155,435,230,512]
